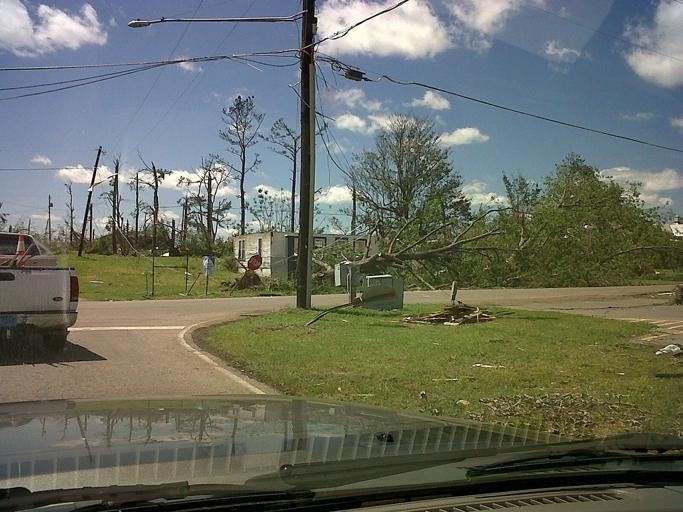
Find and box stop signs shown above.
[247,254,262,270]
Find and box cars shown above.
[0,394,683,512]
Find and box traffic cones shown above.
[16,235,27,267]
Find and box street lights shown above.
[89,173,119,255]
[127,10,318,309]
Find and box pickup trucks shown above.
[1,233,79,355]
